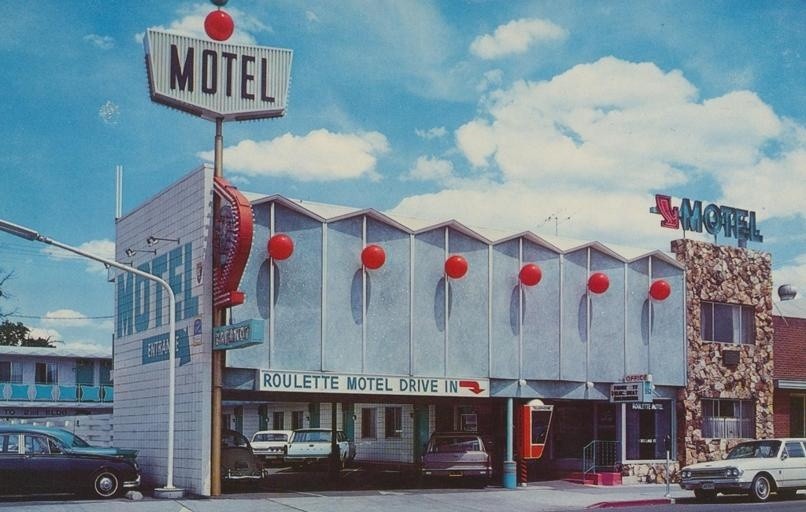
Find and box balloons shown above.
[518,263,544,287]
[266,234,294,261]
[650,279,671,301]
[211,0,228,7]
[588,272,610,294]
[444,255,468,280]
[204,10,234,41]
[361,244,386,270]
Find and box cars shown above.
[679,436,806,502]
[221,428,351,490]
[0,425,141,498]
[419,430,494,489]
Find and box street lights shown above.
[1,219,187,498]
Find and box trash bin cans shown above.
[503,461,516,488]
